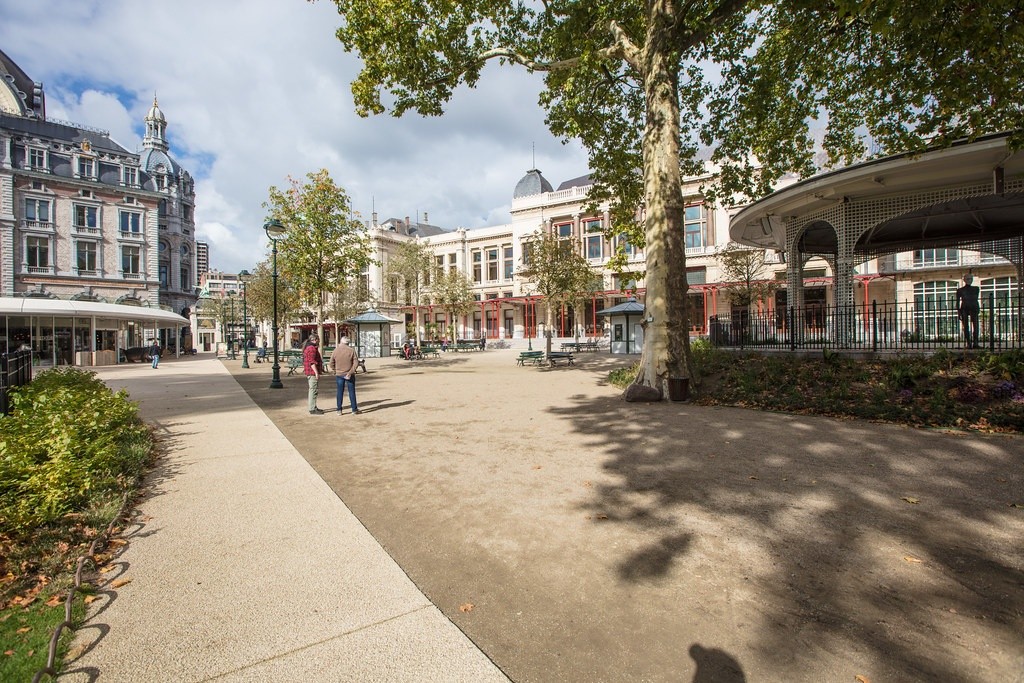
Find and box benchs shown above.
[515,342,601,368]
[394,343,478,362]
[257,350,367,376]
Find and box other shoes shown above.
[351,410,362,415]
[337,410,342,415]
[310,409,324,415]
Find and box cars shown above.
[15,329,103,360]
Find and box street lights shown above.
[227,289,238,360]
[263,219,286,389]
[221,301,229,342]
[238,269,251,368]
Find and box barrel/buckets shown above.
[666,376,689,401]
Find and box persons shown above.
[302,332,322,415]
[152,342,161,369]
[956,273,982,349]
[256,346,265,363]
[329,337,362,416]
